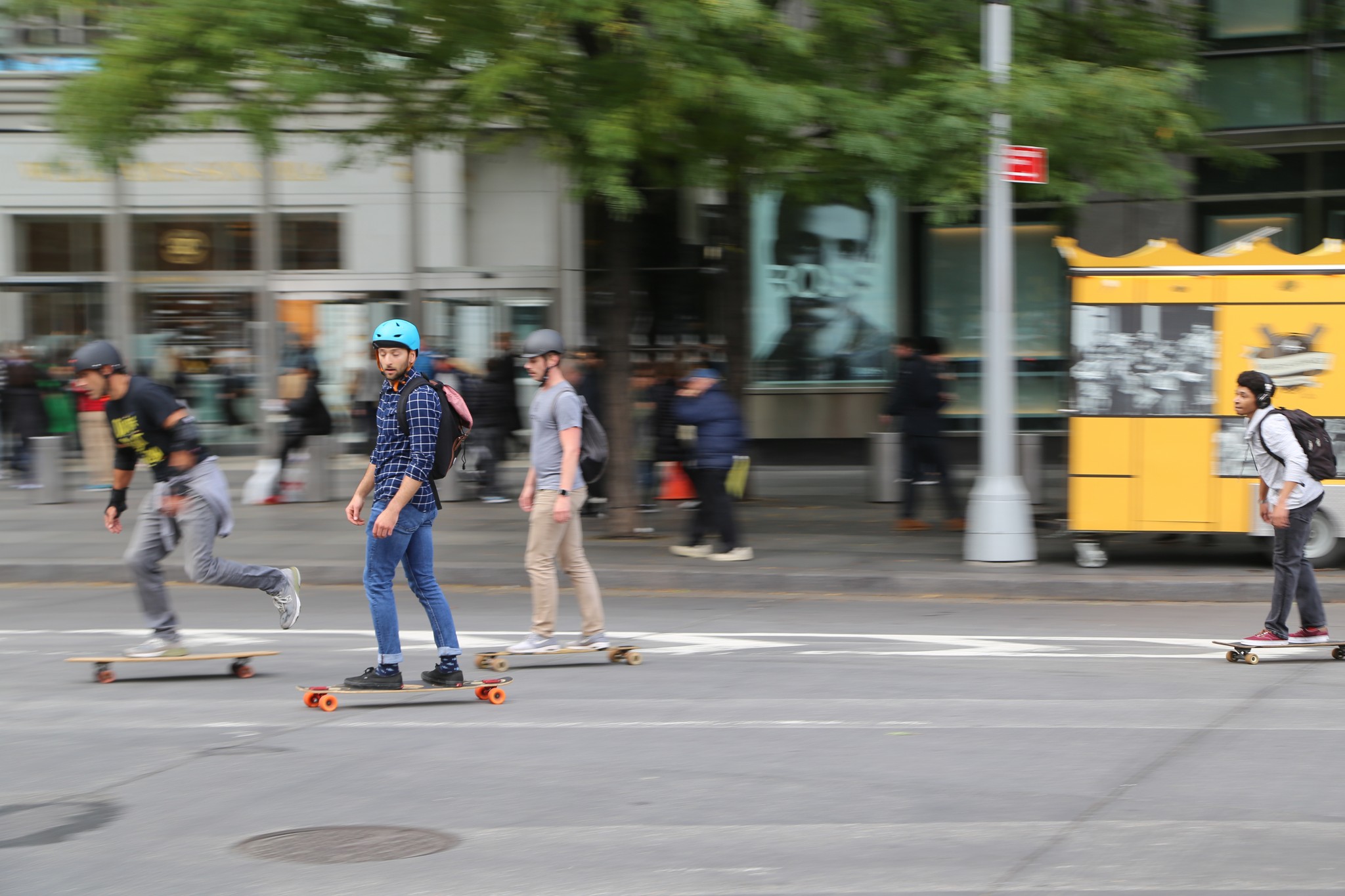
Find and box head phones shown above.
[1254,372,1273,408]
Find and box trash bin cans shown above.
[866,431,906,501]
[29,437,70,503]
[1015,434,1045,504]
[301,436,336,502]
[434,466,479,501]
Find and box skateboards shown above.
[295,676,514,712]
[472,644,645,672]
[62,648,283,684]
[1211,637,1345,664]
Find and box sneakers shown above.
[122,637,188,658]
[506,633,559,653]
[271,566,301,630]
[344,666,404,690]
[1288,628,1330,644]
[1240,629,1289,646]
[566,633,609,650]
[421,663,464,688]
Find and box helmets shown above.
[372,319,421,357]
[69,339,125,376]
[519,329,565,358]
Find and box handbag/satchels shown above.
[651,460,700,500]
[722,454,750,500]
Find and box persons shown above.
[0,320,968,562]
[507,328,609,652]
[68,340,302,659]
[1233,370,1329,646]
[343,318,465,690]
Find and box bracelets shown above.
[1258,499,1268,504]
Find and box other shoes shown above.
[670,542,712,557]
[708,547,753,562]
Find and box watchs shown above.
[558,489,572,497]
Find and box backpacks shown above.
[1258,406,1338,482]
[550,387,610,486]
[396,372,473,511]
